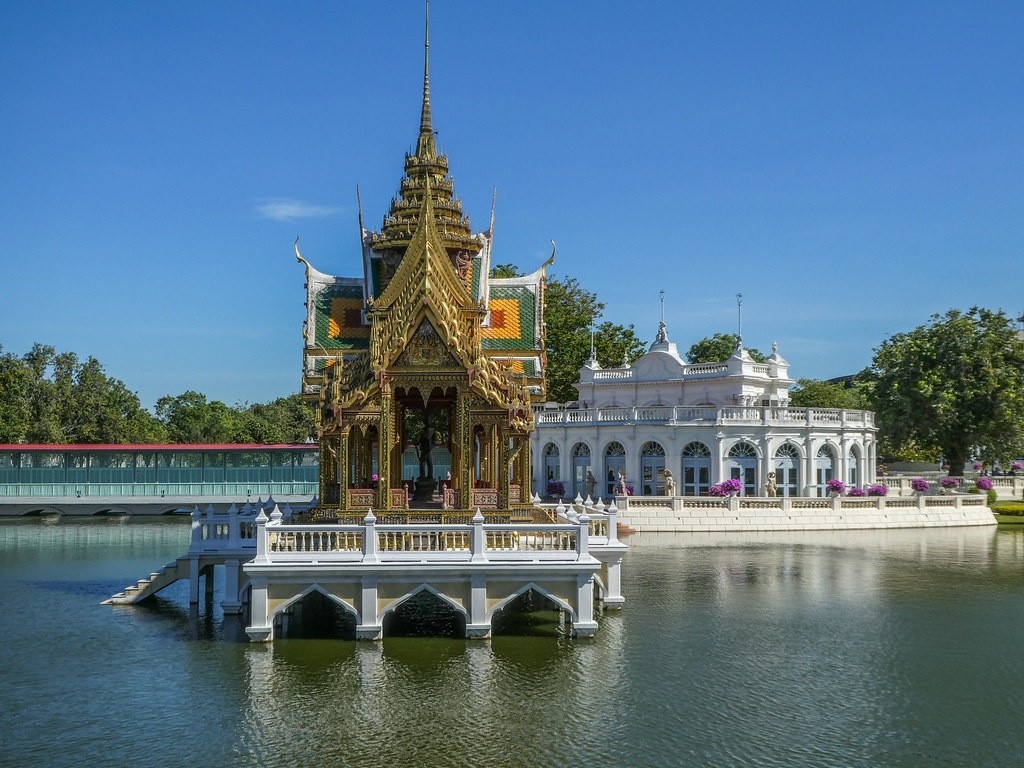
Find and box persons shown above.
[419,426,435,479]
[587,471,595,494]
[662,468,673,496]
[767,472,777,497]
[656,321,668,341]
[618,473,625,493]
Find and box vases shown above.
[551,494,559,498]
[978,489,989,495]
[832,492,840,498]
[948,488,955,494]
[729,491,738,497]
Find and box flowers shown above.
[867,484,888,496]
[940,478,960,489]
[709,478,742,497]
[626,485,634,495]
[826,480,845,498]
[912,479,928,493]
[546,480,566,496]
[975,479,993,491]
[848,489,865,497]
